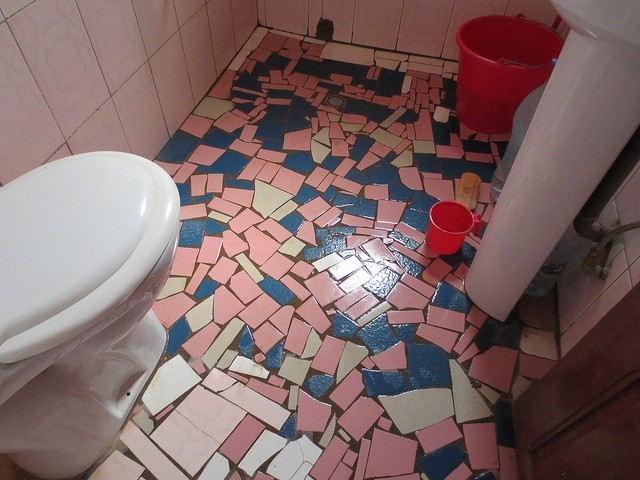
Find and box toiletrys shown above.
[457,172,482,208]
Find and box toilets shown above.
[0,150,180,480]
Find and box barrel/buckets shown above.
[455,13,564,135]
[490,59,557,203]
